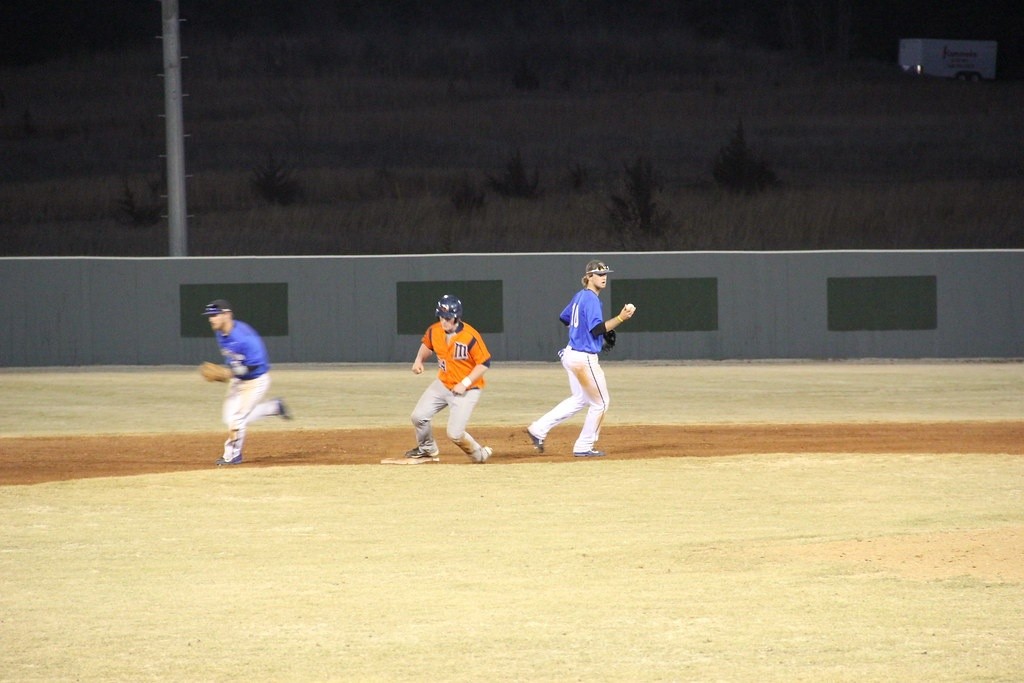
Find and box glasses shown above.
[205,304,223,312]
[589,266,610,273]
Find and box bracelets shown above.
[461,377,472,387]
[618,316,624,322]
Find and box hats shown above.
[202,299,232,317]
[586,261,614,275]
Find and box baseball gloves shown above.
[601,329,617,356]
[200,360,231,384]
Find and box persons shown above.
[527,260,636,457]
[405,295,493,463]
[200,300,294,465]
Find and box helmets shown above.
[435,296,463,322]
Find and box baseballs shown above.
[626,303,634,310]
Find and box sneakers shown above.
[484,446,492,456]
[527,431,544,454]
[573,448,605,458]
[406,447,438,459]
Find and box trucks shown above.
[896,37,999,83]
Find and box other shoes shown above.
[216,454,242,466]
[278,400,291,420]
